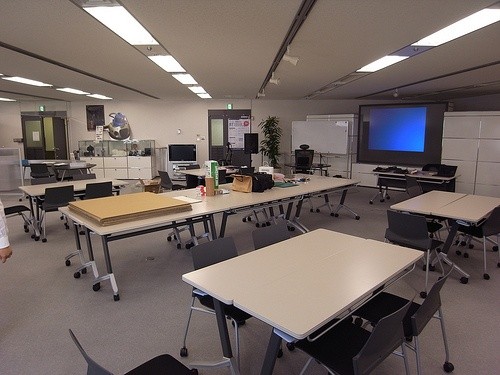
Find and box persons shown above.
[0,201,13,264]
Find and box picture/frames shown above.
[86,105,105,130]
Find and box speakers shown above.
[244,133,259,154]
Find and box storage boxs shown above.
[138,178,161,193]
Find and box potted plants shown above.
[258,115,283,173]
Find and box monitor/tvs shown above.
[168,144,197,161]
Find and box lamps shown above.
[283,44,301,66]
[394,89,400,98]
[269,71,281,85]
[258,89,265,98]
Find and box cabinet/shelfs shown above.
[441,111,500,197]
[78,140,156,180]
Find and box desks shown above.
[18,178,129,242]
[369,170,462,205]
[58,174,360,300]
[182,228,424,375]
[176,165,239,186]
[433,194,500,259]
[24,162,97,183]
[389,190,467,235]
[283,163,331,175]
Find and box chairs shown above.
[4,145,500,375]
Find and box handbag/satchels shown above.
[248,172,274,192]
[258,161,274,182]
[230,174,252,193]
